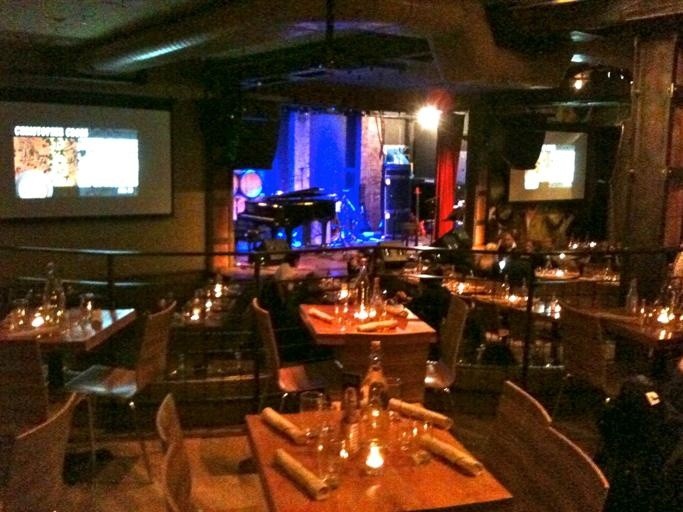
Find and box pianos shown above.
[232,187,335,266]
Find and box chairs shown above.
[155,393,271,510]
[63,299,178,469]
[180,248,682,511]
[2,392,77,510]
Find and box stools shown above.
[393,220,419,249]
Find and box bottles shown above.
[543,233,617,279]
[37,262,70,328]
[446,261,560,315]
[360,341,391,418]
[622,276,683,328]
[333,258,390,321]
[185,270,229,316]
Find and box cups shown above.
[77,292,94,316]
[12,297,30,320]
[300,392,433,485]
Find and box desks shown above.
[2,305,136,437]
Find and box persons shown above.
[347,214,537,367]
[270,251,305,295]
[607,336,682,512]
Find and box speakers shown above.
[505,113,547,170]
[384,162,413,210]
[371,239,407,266]
[258,238,292,262]
[233,99,281,168]
